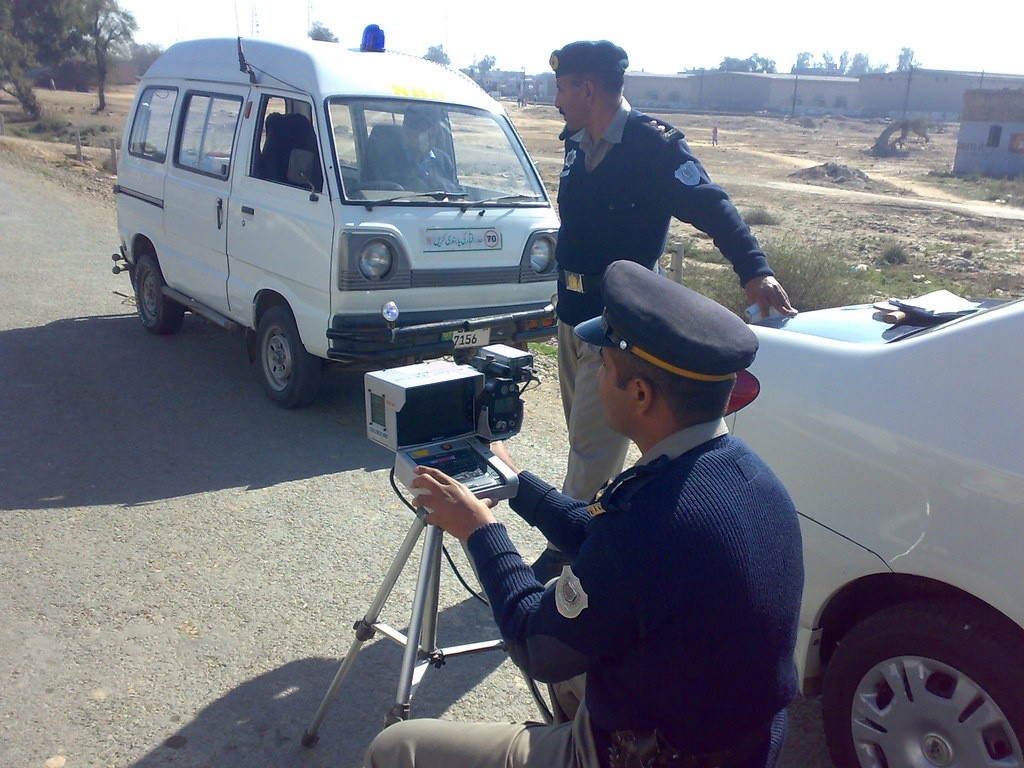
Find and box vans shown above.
[110,21,563,412]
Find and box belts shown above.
[557,268,659,294]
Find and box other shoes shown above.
[530,548,563,588]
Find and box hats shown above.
[403,104,441,134]
[574,262,759,381]
[549,40,629,78]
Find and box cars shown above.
[721,287,1024,768]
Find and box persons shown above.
[712,127,718,147]
[531,40,799,585]
[517,94,537,107]
[368,103,468,201]
[364,260,805,768]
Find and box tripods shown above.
[301,506,553,749]
